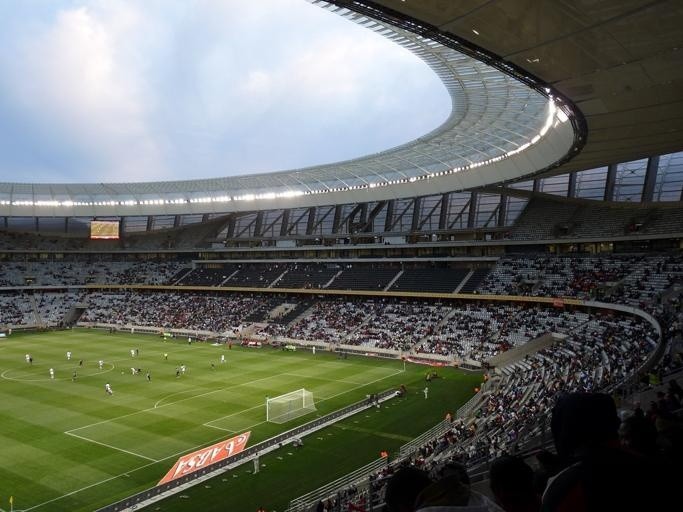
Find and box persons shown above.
[163,336,249,379]
[1,232,682,336]
[26,348,151,394]
[311,323,682,510]
[253,453,259,474]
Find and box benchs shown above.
[0,190,683,512]
